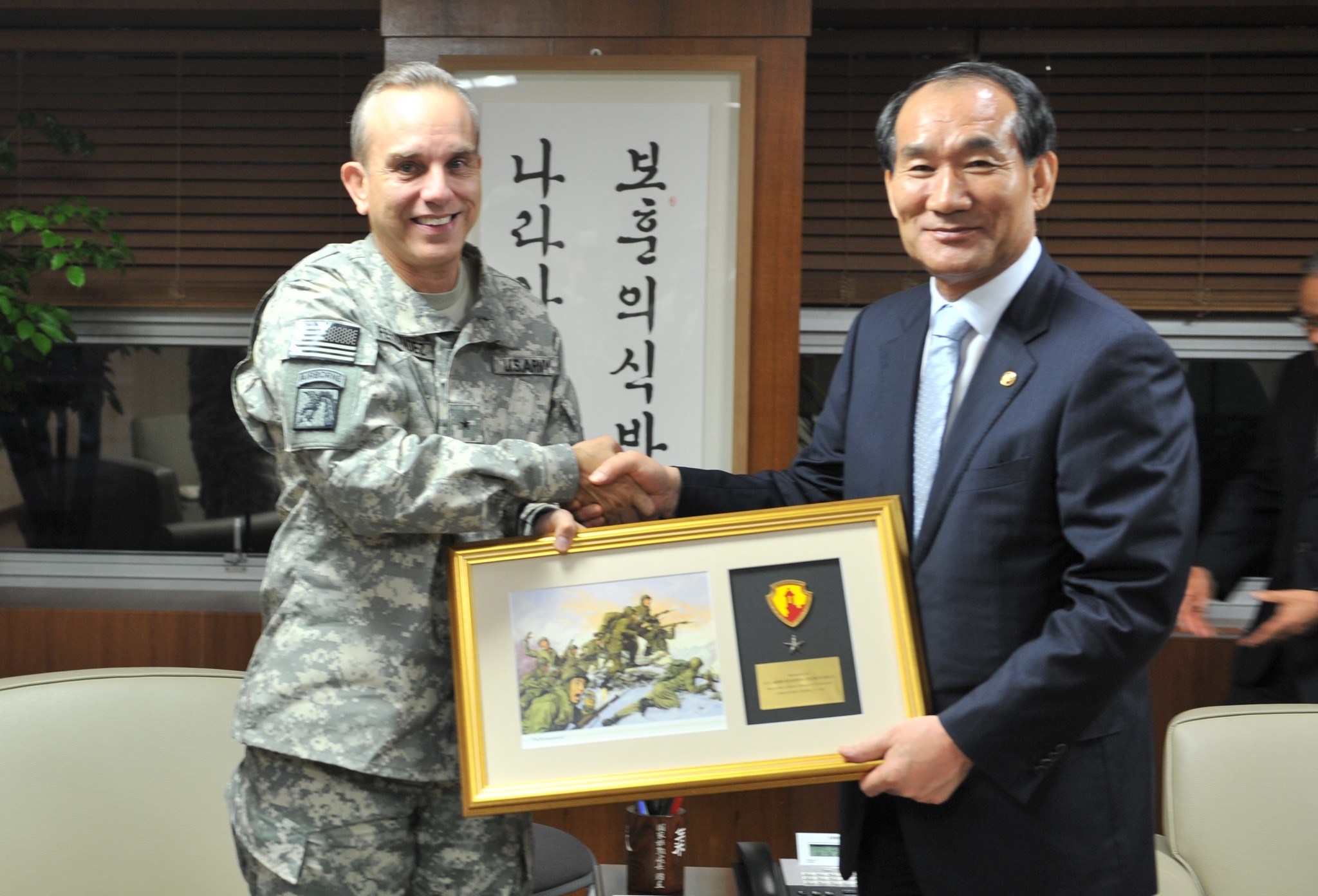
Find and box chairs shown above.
[135,406,205,522]
[0,665,249,896]
[1157,704,1318,896]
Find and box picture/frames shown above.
[447,495,930,819]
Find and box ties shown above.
[912,303,972,541]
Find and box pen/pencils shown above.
[636,798,684,815]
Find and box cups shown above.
[624,804,688,896]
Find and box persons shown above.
[567,62,1201,896]
[1177,258,1318,704]
[226,63,655,896]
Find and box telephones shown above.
[731,832,858,896]
